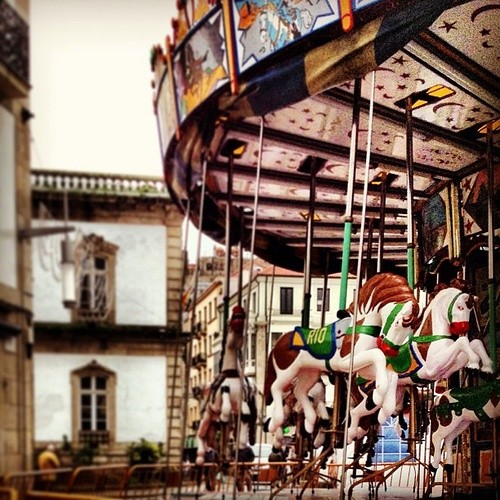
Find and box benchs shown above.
[35,450,263,482]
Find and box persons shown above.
[38,444,60,492]
[222,431,296,495]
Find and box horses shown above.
[195,305,256,465]
[429,375,500,473]
[278,279,495,452]
[262,273,416,451]
